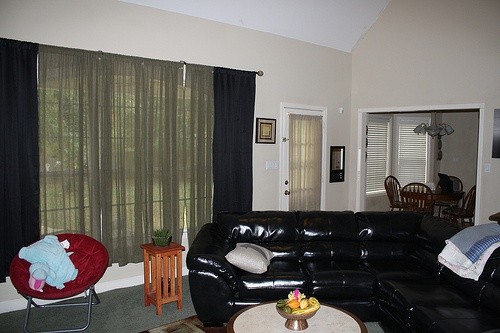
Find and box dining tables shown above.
[399,191,465,218]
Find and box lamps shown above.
[413,113,456,139]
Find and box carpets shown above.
[137,313,228,333]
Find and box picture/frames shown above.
[256,117,277,145]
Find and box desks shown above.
[139,241,185,315]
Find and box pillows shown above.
[224,243,275,274]
[18,235,78,289]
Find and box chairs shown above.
[435,175,463,220]
[384,175,418,211]
[399,182,435,216]
[442,184,476,230]
[9,233,109,333]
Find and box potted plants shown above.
[151,227,178,247]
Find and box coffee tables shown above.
[227,297,368,333]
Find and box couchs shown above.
[186,208,500,333]
